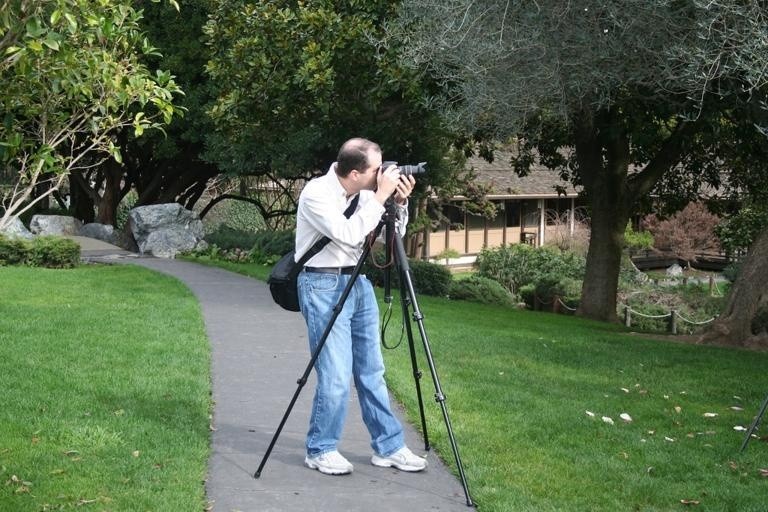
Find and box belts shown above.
[302,265,359,274]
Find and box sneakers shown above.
[303,448,354,475]
[370,444,429,472]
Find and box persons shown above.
[294,137,429,475]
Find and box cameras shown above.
[380,162,428,198]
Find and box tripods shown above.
[255,197,472,508]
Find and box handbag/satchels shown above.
[270,248,301,312]
